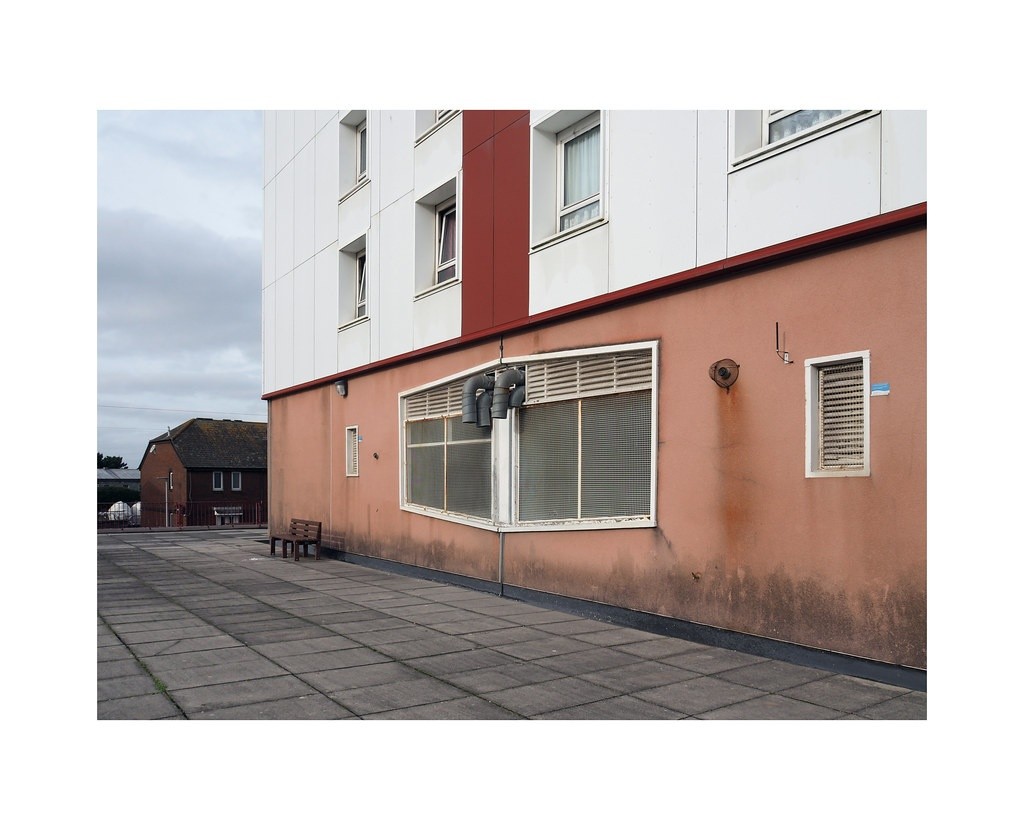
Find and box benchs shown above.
[271,518,322,561]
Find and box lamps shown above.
[335,380,347,397]
[709,359,739,394]
